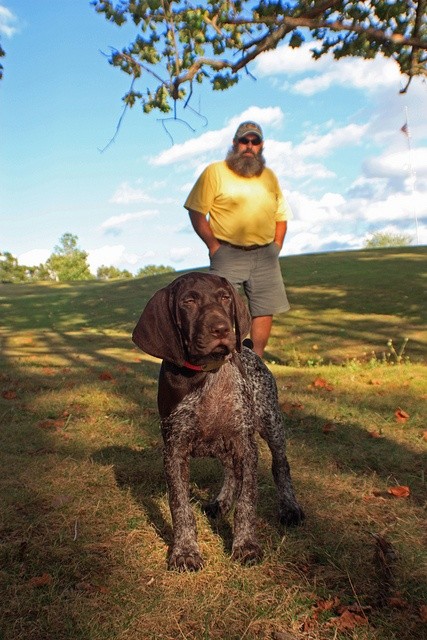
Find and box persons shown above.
[183,121,290,359]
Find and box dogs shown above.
[131,271,305,573]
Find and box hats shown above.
[236,122,263,139]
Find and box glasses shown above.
[239,138,261,145]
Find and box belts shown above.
[217,239,269,251]
